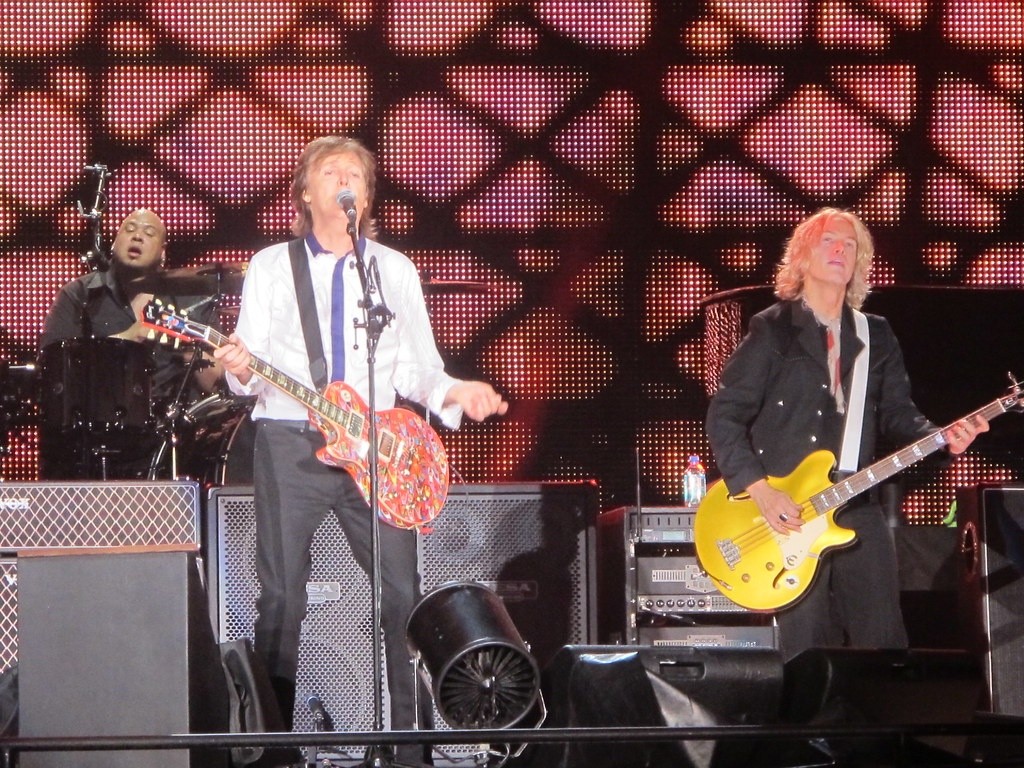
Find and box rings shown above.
[779,512,789,522]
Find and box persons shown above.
[705,206,989,649]
[37,210,223,481]
[213,136,508,768]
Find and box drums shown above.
[188,392,257,484]
[39,337,152,446]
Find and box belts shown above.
[259,418,322,431]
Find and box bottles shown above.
[684,455,706,508]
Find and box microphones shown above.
[305,695,324,720]
[88,243,110,272]
[337,189,357,217]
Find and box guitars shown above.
[694,368,1023,612]
[141,300,450,525]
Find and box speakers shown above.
[0,481,391,768]
[415,480,1024,768]
